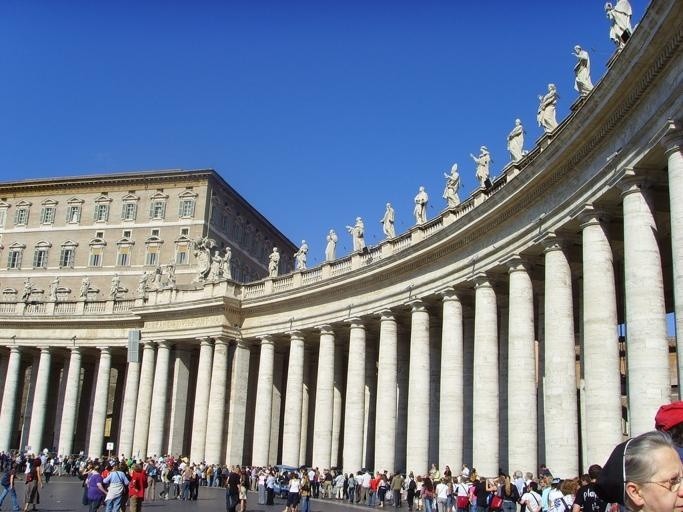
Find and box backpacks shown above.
[560,497,572,512]
[0,473,10,488]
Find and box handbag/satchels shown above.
[25,473,32,481]
[490,496,502,510]
[301,485,307,490]
[457,496,468,509]
[123,487,128,494]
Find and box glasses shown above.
[626,477,682,493]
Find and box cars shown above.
[270,465,298,499]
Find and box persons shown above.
[571,45,594,96]
[269,247,279,278]
[469,146,490,187]
[345,217,365,256]
[199,461,405,512]
[326,229,338,263]
[0,448,63,512]
[507,118,524,162]
[537,83,559,133]
[442,163,460,208]
[379,203,396,239]
[413,186,428,225]
[605,0,632,52]
[292,239,308,271]
[405,403,683,512]
[18,245,233,302]
[63,454,201,512]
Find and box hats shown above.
[654,401,682,432]
[592,438,633,507]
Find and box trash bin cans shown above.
[264,489,273,505]
